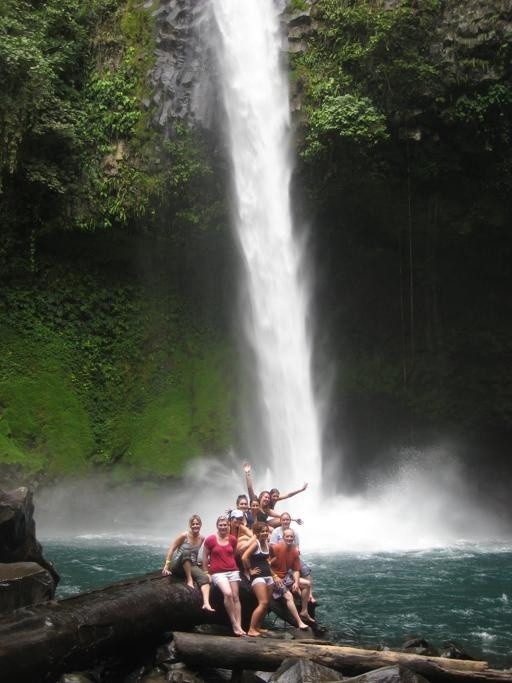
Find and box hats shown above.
[230,509,243,518]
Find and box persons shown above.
[162,462,316,636]
